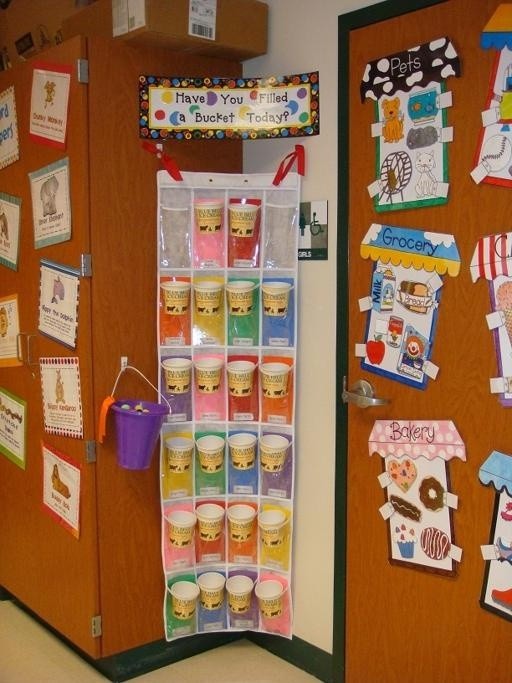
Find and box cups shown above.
[225,280,255,315]
[160,281,191,315]
[261,362,291,399]
[162,358,192,394]
[166,437,194,473]
[198,572,226,611]
[193,280,223,316]
[260,434,289,473]
[170,581,201,621]
[261,282,292,316]
[194,201,224,233]
[196,358,223,394]
[196,435,226,473]
[195,503,225,541]
[254,580,284,620]
[258,509,287,549]
[226,575,253,614]
[228,203,259,237]
[167,510,195,548]
[227,504,256,543]
[227,360,256,396]
[229,433,257,470]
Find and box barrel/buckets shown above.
[109,364,171,473]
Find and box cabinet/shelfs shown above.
[0,37,240,660]
